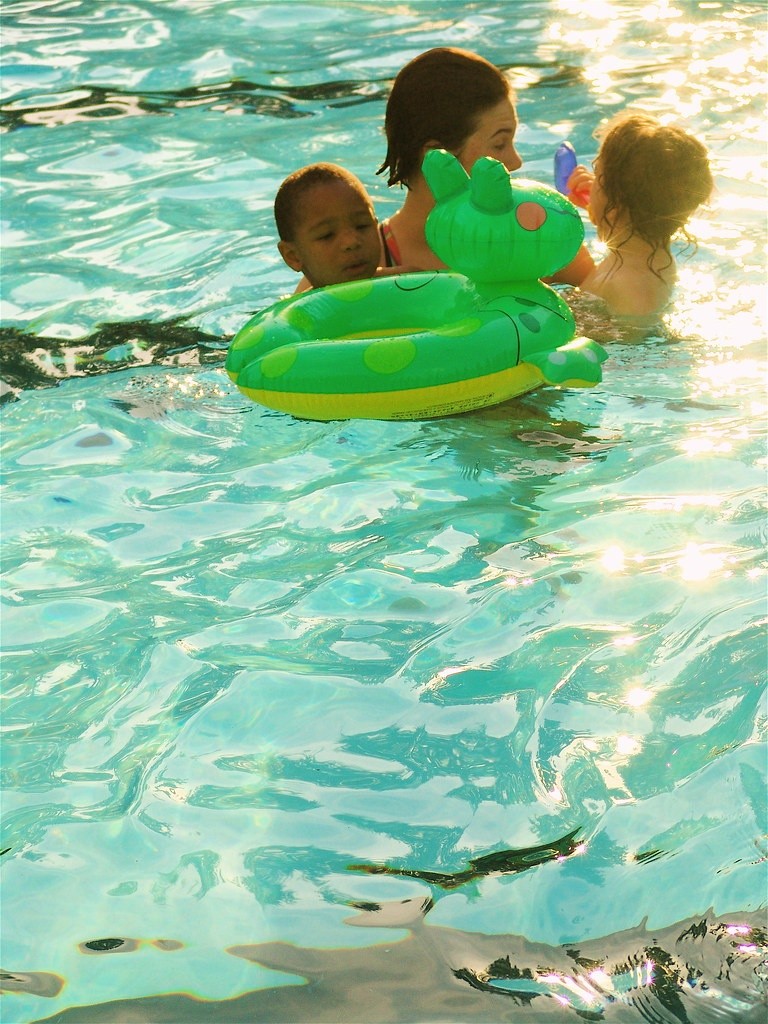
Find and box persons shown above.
[565,112,713,342]
[274,161,425,297]
[290,47,597,288]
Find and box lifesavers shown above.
[223,146,611,421]
